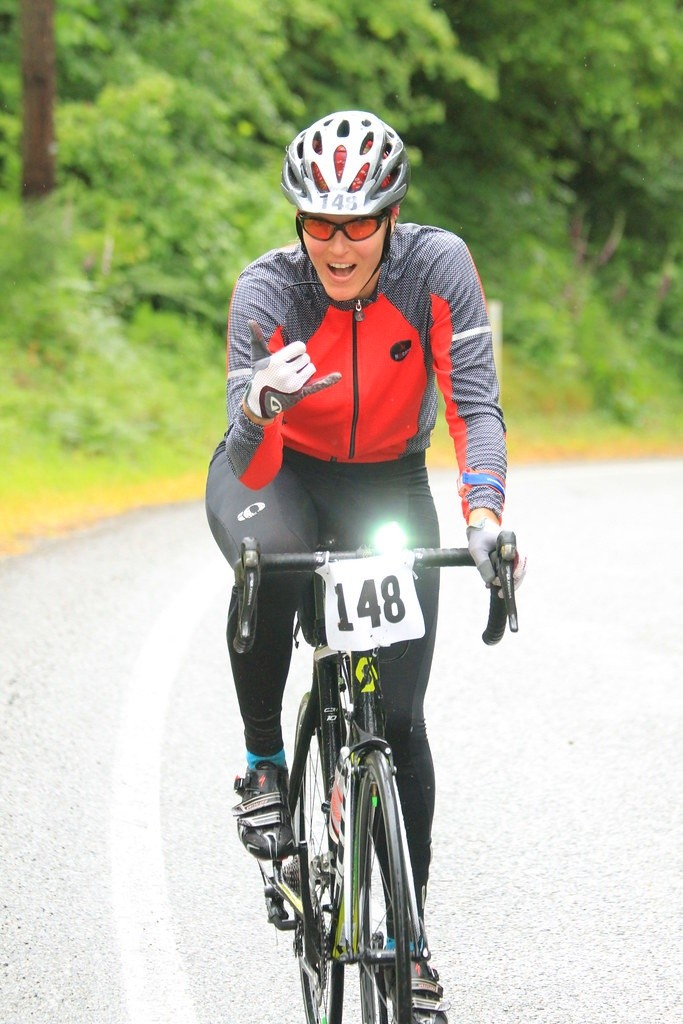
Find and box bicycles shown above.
[231,520,520,1024]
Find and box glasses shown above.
[296,211,394,242]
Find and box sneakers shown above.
[232,758,297,861]
[379,941,451,1024]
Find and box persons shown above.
[206,111,524,1023]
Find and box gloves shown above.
[244,320,343,421]
[466,518,528,600]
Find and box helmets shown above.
[280,111,411,216]
